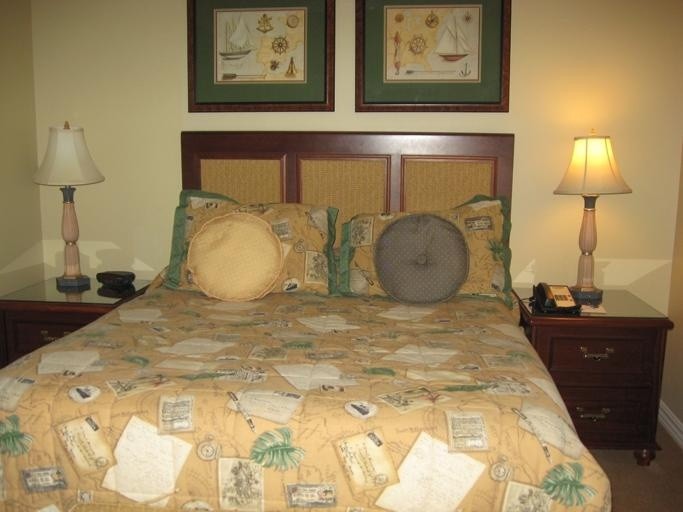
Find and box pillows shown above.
[372,213,470,307]
[163,189,338,295]
[187,213,283,302]
[339,194,512,298]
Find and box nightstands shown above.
[0,275,154,371]
[514,286,673,466]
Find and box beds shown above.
[0,130,570,512]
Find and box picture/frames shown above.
[186,1,337,113]
[352,5,512,115]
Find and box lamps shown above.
[34,121,104,290]
[551,129,632,302]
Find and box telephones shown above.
[528,282,581,316]
